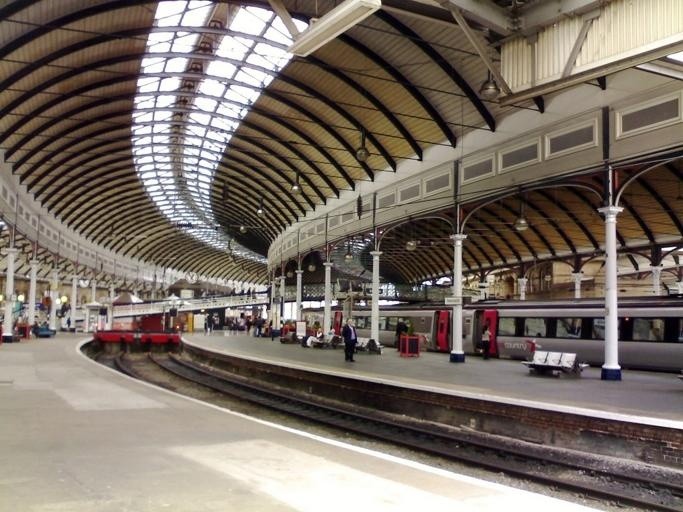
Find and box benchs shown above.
[522,351,589,379]
[354,336,382,355]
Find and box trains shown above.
[301,297,683,373]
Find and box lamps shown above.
[479,58,500,100]
[355,127,369,162]
[511,195,529,232]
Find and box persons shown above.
[396,317,408,351]
[481,325,492,359]
[67,317,71,328]
[316,328,324,341]
[245,316,265,337]
[342,319,358,362]
[207,313,215,333]
